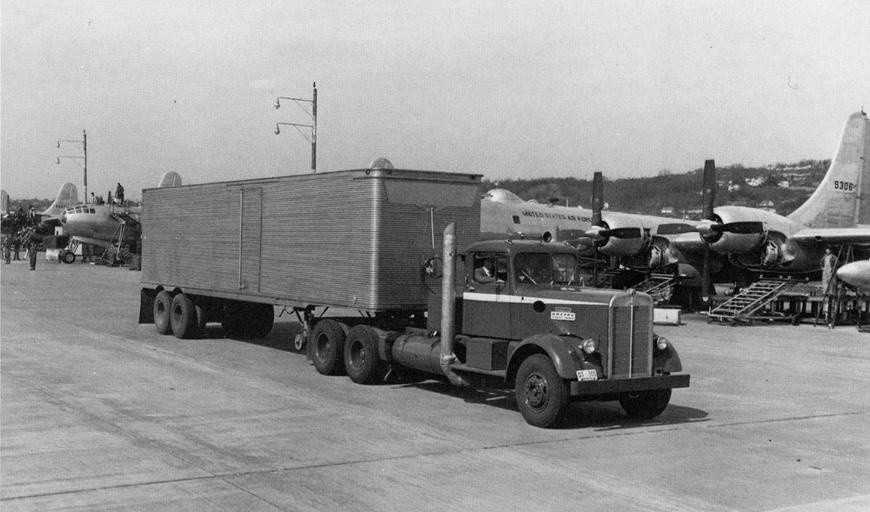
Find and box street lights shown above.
[274,81,317,173]
[55,128,88,205]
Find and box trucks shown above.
[134,168,691,428]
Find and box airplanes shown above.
[1,170,183,265]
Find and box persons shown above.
[0,204,58,271]
[90,182,124,207]
[515,255,541,284]
[818,247,839,296]
[473,259,505,284]
